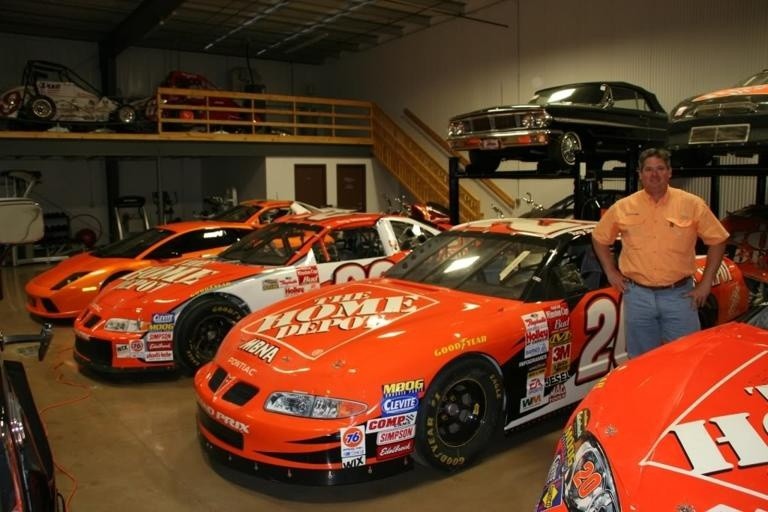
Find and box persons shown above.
[591,148,730,358]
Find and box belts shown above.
[628,275,688,291]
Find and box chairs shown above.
[340,231,361,260]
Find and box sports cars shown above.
[0,57,138,131]
[123,69,261,132]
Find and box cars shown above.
[446,80,669,171]
[664,69,767,166]
